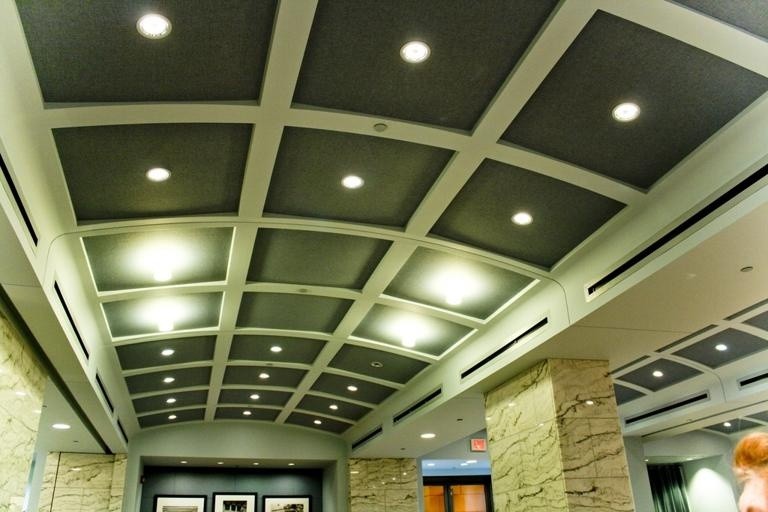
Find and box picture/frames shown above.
[262,495,313,512]
[212,492,257,512]
[153,494,207,512]
